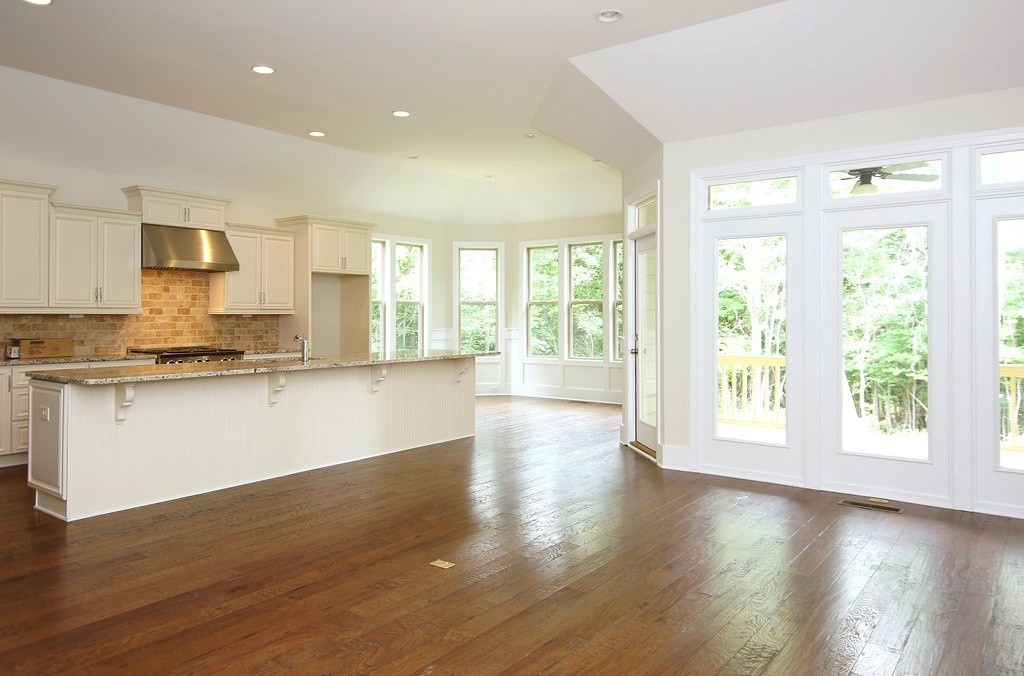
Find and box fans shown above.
[831,162,938,183]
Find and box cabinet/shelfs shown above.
[0,365,31,469]
[0,176,374,315]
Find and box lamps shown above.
[850,173,877,197]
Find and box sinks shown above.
[238,357,329,363]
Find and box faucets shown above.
[291,330,309,362]
